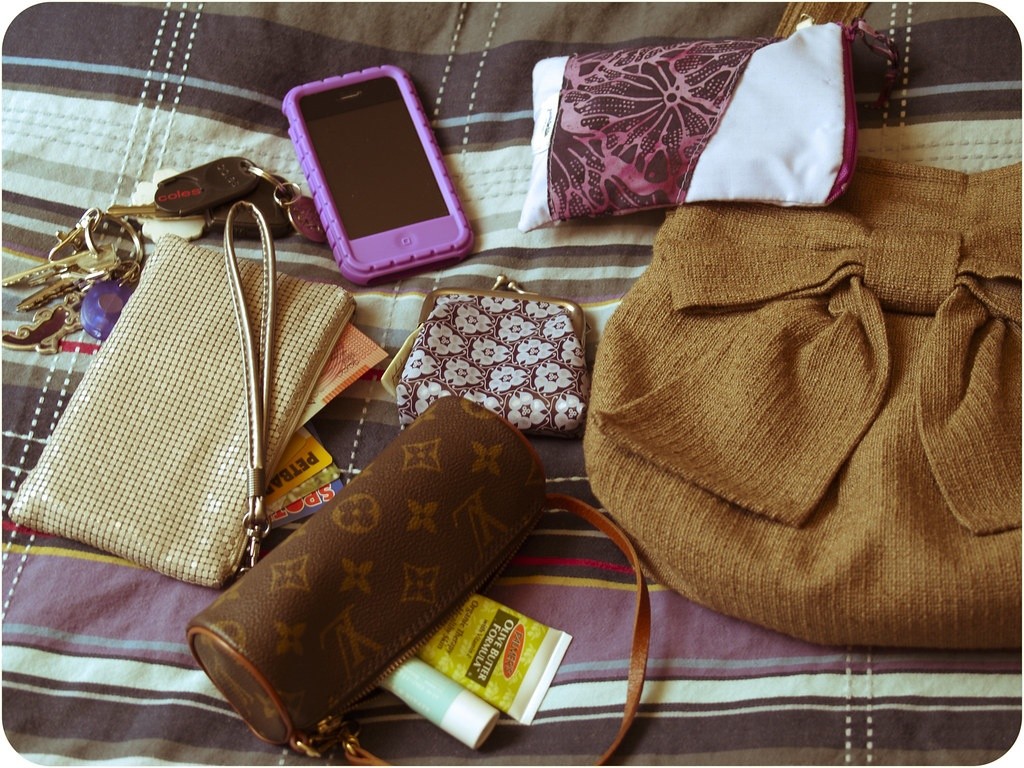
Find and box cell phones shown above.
[281,65,475,288]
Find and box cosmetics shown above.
[380,593,574,750]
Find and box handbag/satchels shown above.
[12,241,355,588]
[519,16,904,233]
[179,397,649,768]
[398,275,592,437]
[579,2,1023,657]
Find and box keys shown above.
[106,157,289,239]
[2,240,121,313]
